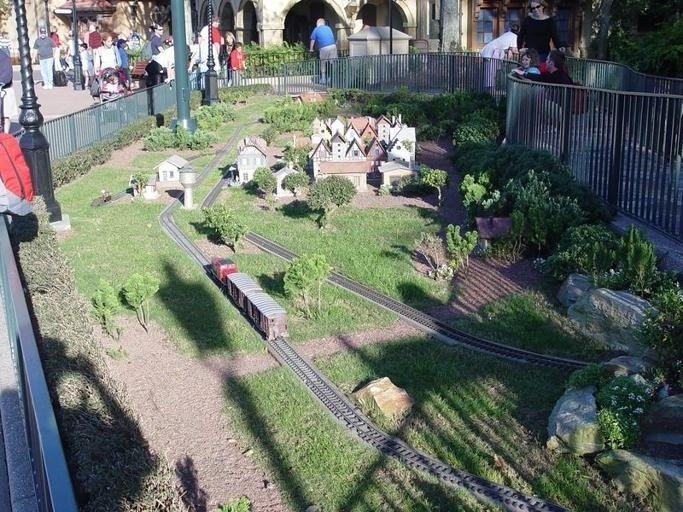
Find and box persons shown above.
[480,24,521,99]
[0,31,10,50]
[0,50,14,87]
[0,118,34,216]
[515,1,566,61]
[520,49,541,77]
[511,50,574,135]
[309,18,339,84]
[31,17,245,100]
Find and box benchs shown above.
[128,61,149,77]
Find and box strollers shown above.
[99,68,128,123]
